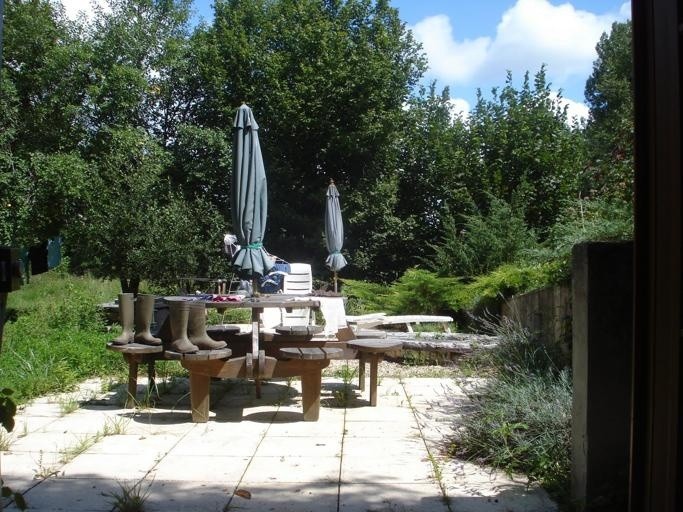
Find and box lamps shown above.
[278,261,314,327]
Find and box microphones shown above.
[109,324,401,424]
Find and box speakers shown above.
[134,292,162,346]
[187,300,228,350]
[167,301,199,354]
[112,293,135,345]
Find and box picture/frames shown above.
[103,294,340,421]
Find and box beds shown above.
[228,101,277,321]
[324,178,348,292]
